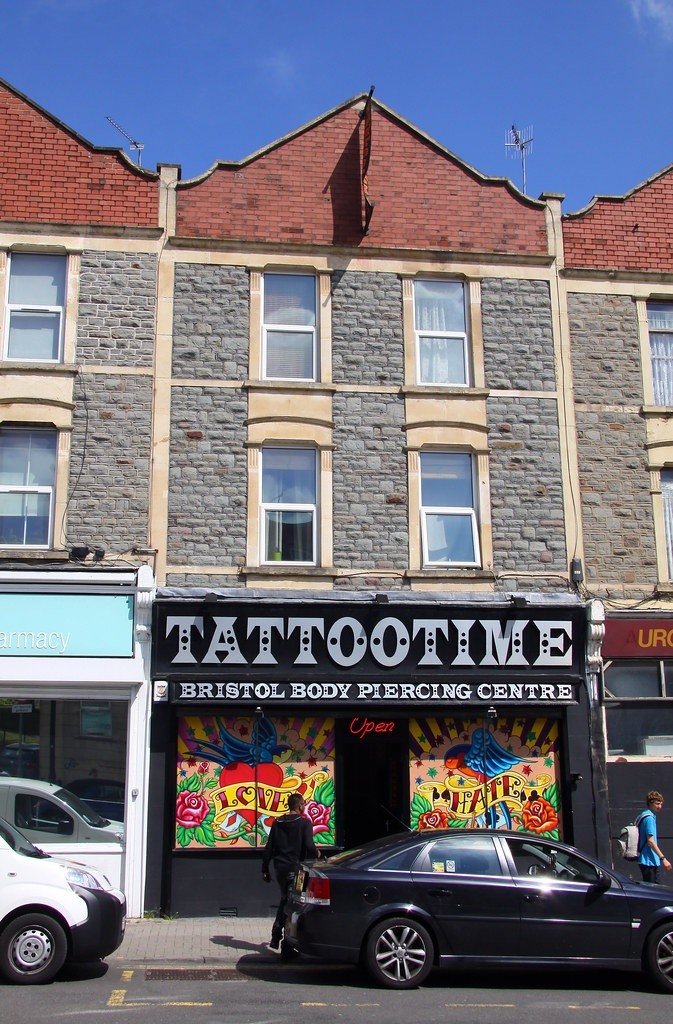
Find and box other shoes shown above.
[269,936,280,950]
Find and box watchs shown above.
[660,856,666,861]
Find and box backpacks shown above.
[617,814,655,861]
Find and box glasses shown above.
[302,800,306,805]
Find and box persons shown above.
[634,790,672,884]
[261,793,322,950]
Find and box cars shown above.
[0,817,126,986]
[0,777,128,897]
[282,827,672,992]
